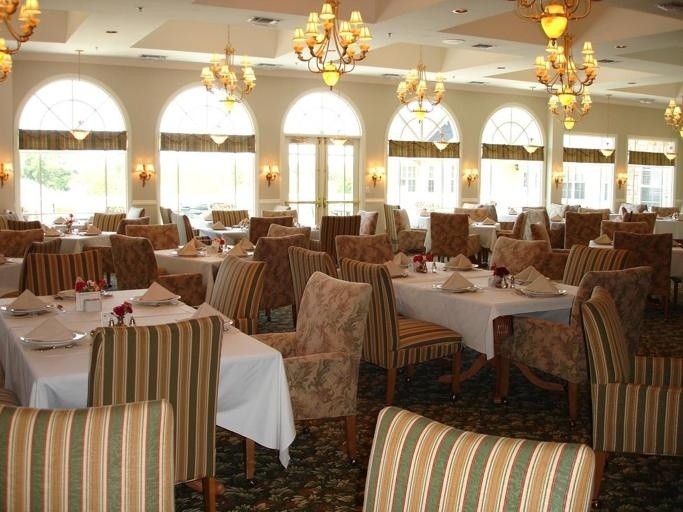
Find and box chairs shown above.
[576,281,680,501]
[81,314,224,511]
[236,268,373,481]
[0,397,176,512]
[355,405,606,512]
[0,199,683,424]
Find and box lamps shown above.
[393,44,448,129]
[663,147,682,164]
[549,168,570,189]
[197,23,255,117]
[0,0,46,87]
[431,133,451,153]
[69,123,94,144]
[662,94,682,139]
[512,0,603,132]
[598,140,619,167]
[259,161,282,191]
[209,134,232,149]
[365,164,390,190]
[458,161,485,191]
[134,161,157,190]
[291,0,374,91]
[613,168,633,191]
[326,135,352,150]
[1,159,15,190]
[520,137,541,156]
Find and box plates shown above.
[433,282,480,292]
[445,264,479,271]
[520,289,568,296]
[131,294,182,305]
[176,318,233,327]
[174,244,256,258]
[57,289,105,301]
[19,328,87,348]
[0,302,55,315]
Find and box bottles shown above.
[432,262,436,272]
[129,319,135,327]
[488,276,516,289]
[408,260,428,273]
[108,319,114,327]
